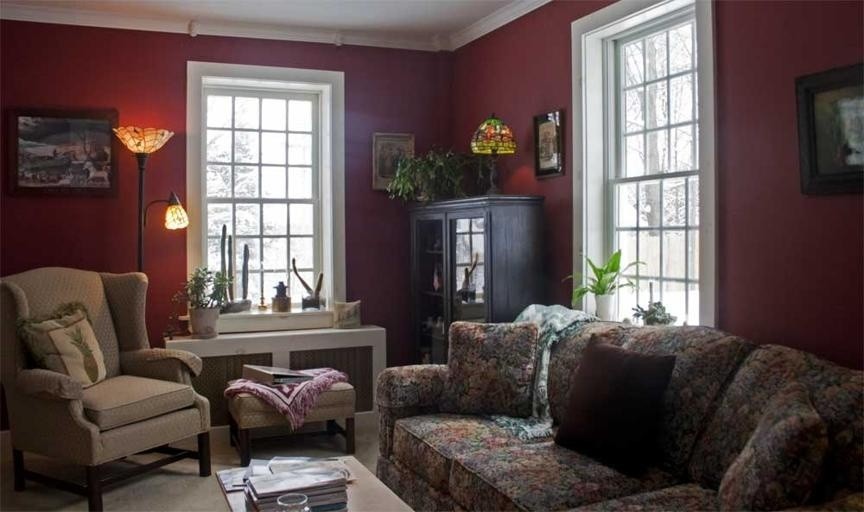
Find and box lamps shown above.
[0,266,212,512]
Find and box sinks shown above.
[373,305,863,510]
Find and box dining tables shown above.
[386,148,480,204]
[169,267,233,339]
[563,250,646,322]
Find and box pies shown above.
[260,263,264,306]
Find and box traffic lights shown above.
[554,334,676,473]
[14,302,106,388]
[441,321,537,417]
[717,382,828,509]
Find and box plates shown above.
[163,325,388,433]
[409,193,545,364]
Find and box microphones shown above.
[226,380,356,464]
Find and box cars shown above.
[277,492,311,512]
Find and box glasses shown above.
[470,116,517,194]
[113,125,189,271]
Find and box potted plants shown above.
[794,63,863,195]
[532,109,564,180]
[2,106,118,197]
[371,131,416,190]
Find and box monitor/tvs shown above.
[217,456,349,512]
